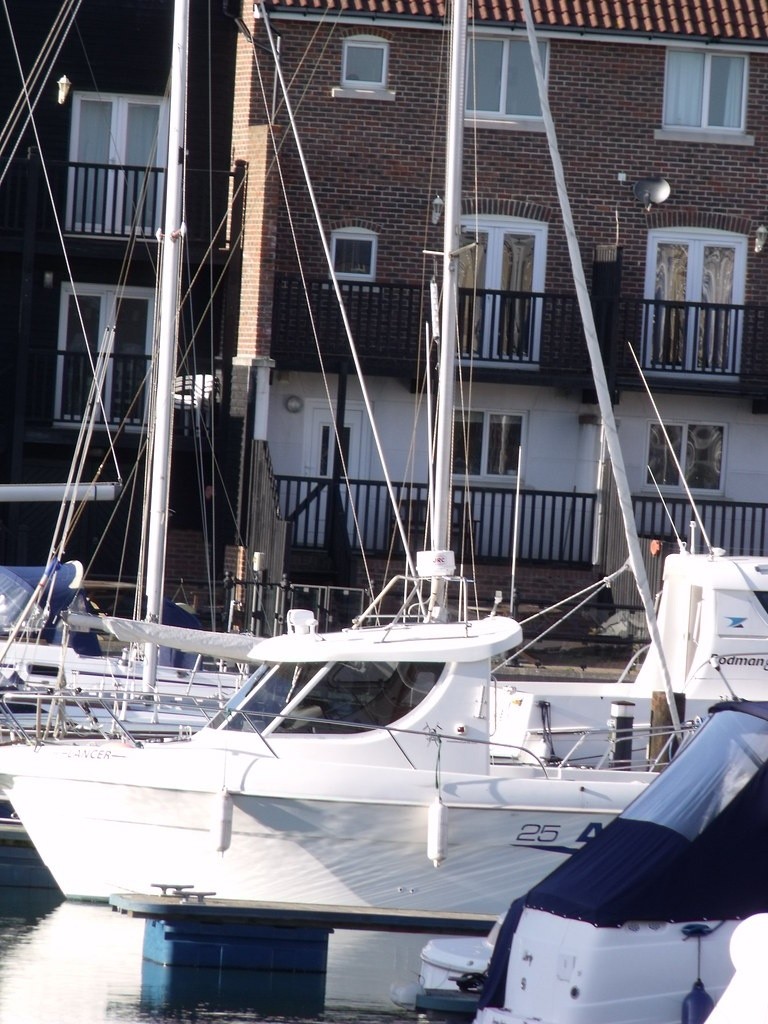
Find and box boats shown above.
[419,690,768,1024]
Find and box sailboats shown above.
[0,0,768,913]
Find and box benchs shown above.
[390,500,474,557]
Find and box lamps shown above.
[431,196,444,225]
[755,225,768,253]
[57,75,72,104]
